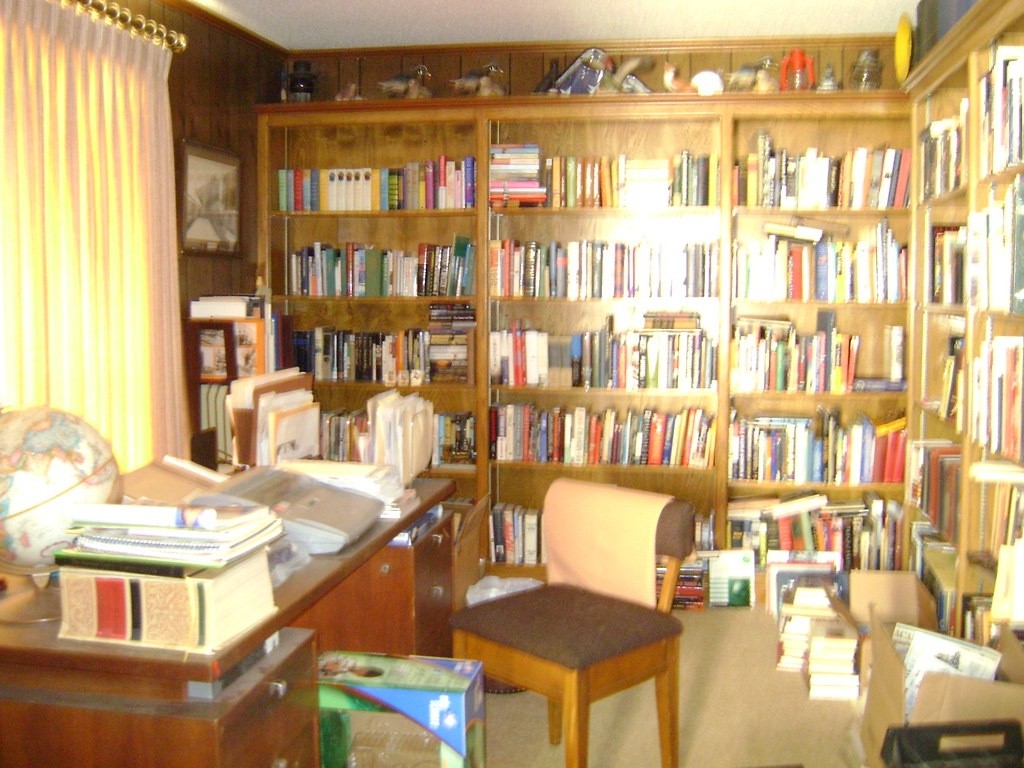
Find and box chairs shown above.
[448,477,695,768]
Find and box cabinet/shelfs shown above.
[254,0,1024,683]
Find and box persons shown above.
[315,653,383,677]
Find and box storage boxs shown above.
[317,650,488,768]
[845,568,1024,768]
[445,492,489,614]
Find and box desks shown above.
[0,457,458,768]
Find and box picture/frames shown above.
[175,135,246,259]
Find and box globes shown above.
[0,403,123,625]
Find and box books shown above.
[56,48,1024,730]
